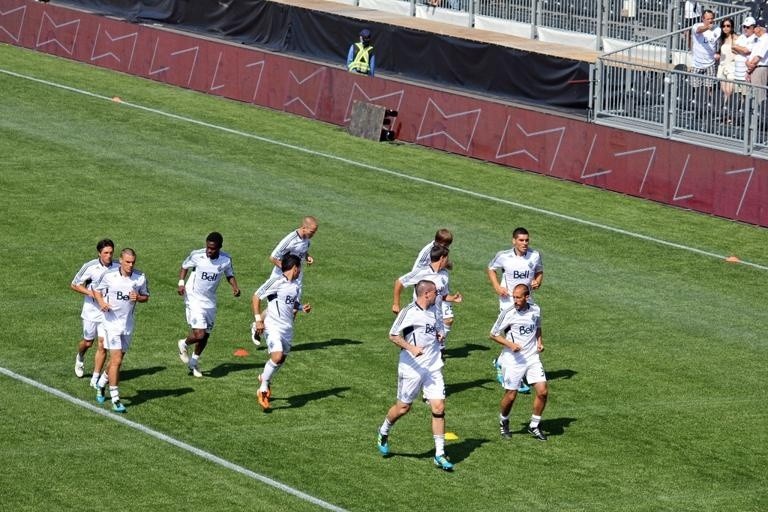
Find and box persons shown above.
[488,227,543,394]
[177,231,240,378]
[71,240,121,388]
[490,284,548,440]
[427,0,440,7]
[252,254,312,412]
[346,29,376,77]
[376,229,462,472]
[683,0,768,137]
[250,216,319,346]
[91,248,151,413]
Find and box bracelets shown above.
[255,314,262,322]
[177,279,185,287]
[296,304,302,311]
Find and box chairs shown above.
[483,0,768,132]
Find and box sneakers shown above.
[499,413,512,440]
[527,425,546,440]
[434,455,453,469]
[493,358,504,384]
[178,339,189,364]
[258,374,271,398]
[75,352,126,412]
[517,380,530,393]
[251,322,261,345]
[378,426,390,454]
[189,363,202,377]
[257,389,271,409]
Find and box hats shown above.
[741,16,768,27]
[360,29,371,36]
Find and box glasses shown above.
[722,25,731,27]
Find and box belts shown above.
[756,65,768,67]
